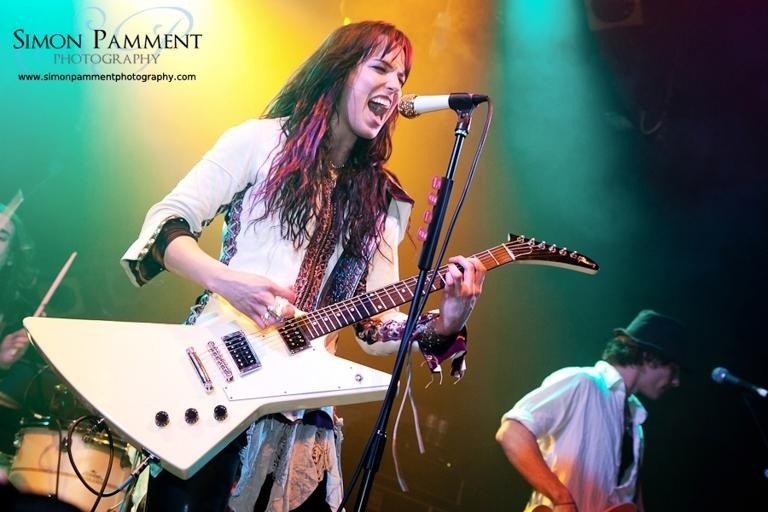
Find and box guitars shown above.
[21,234,599,480]
[531,501,638,511]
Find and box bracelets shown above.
[555,502,575,507]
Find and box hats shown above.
[612,309,693,364]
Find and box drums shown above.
[8,413,134,511]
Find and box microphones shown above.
[709,365,768,397]
[397,93,487,119]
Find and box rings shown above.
[261,313,272,325]
[269,303,285,319]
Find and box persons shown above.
[118,21,485,512]
[0,203,47,512]
[495,308,695,512]
